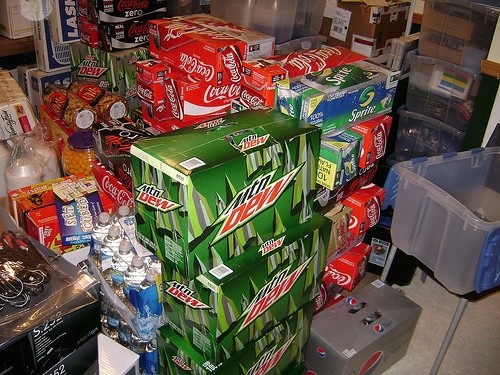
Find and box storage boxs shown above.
[0,0,500,375]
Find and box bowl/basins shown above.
[0,140,62,200]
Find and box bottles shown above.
[62,128,97,180]
[40,84,96,128]
[86,205,161,375]
[67,81,127,121]
